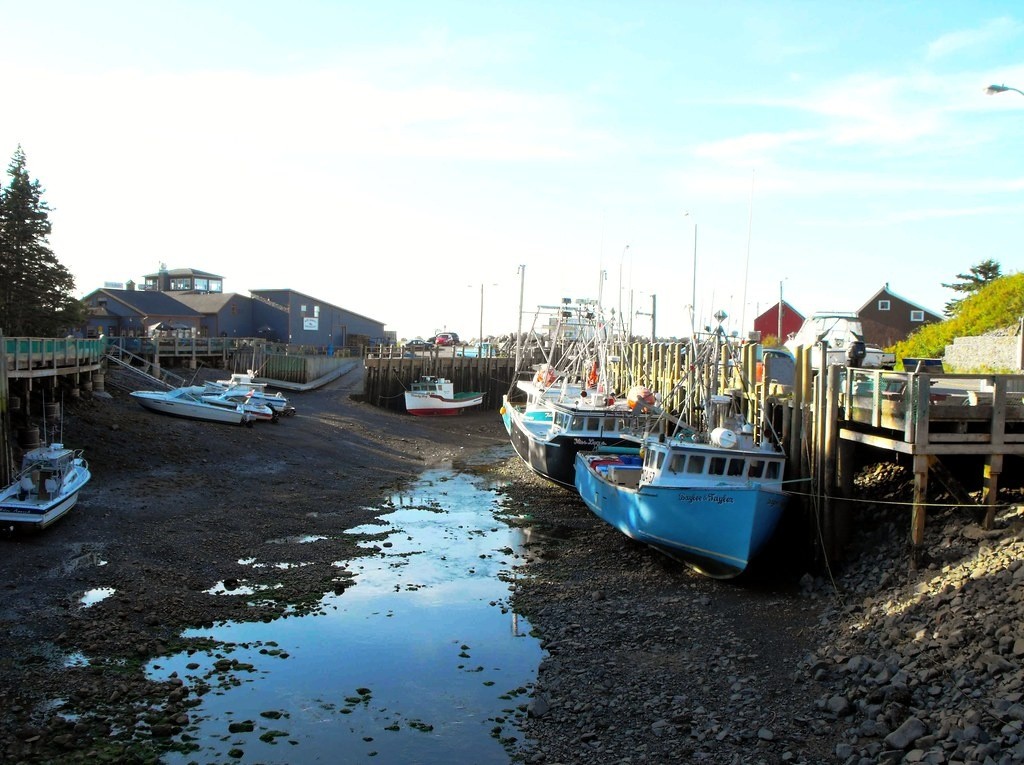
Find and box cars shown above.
[405,340,434,351]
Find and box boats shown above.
[404,375,486,418]
[129,384,274,424]
[793,312,893,371]
[497,281,789,573]
[206,374,297,416]
[0,442,92,526]
